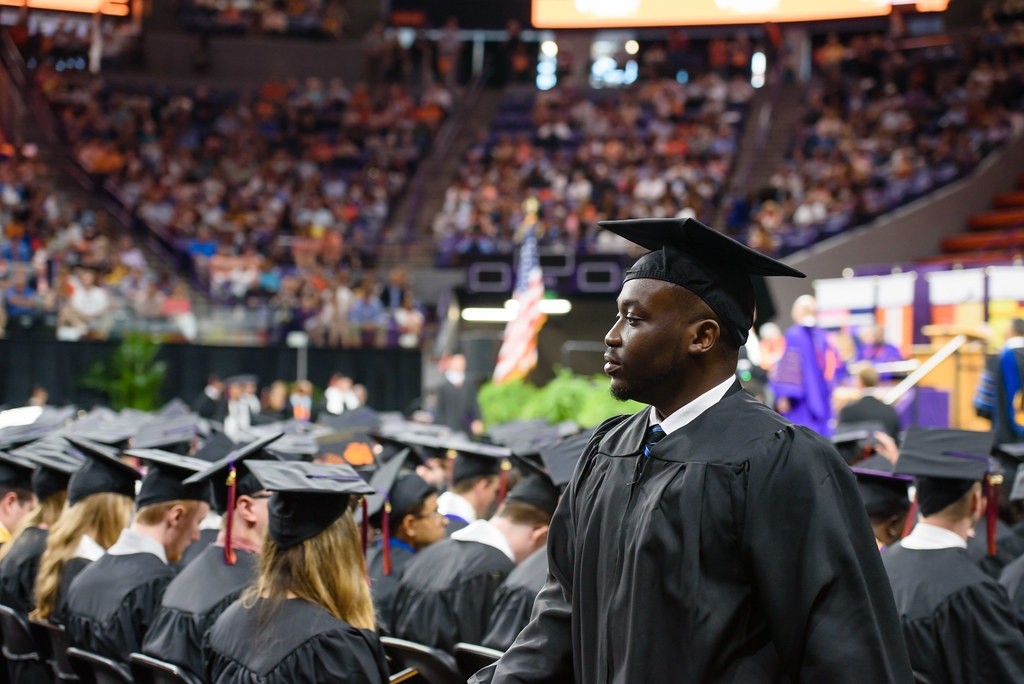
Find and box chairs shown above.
[379,635,506,684]
[0,605,205,684]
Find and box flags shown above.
[491,224,545,383]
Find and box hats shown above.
[849,452,921,540]
[998,440,1024,502]
[599,218,807,348]
[893,424,1002,556]
[0,405,596,551]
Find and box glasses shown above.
[412,503,442,520]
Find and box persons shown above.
[0,76,198,345]
[0,0,429,95]
[480,429,597,653]
[731,32,1024,256]
[828,396,1024,615]
[24,436,143,627]
[883,425,1024,684]
[68,447,214,672]
[0,379,547,636]
[198,461,390,684]
[392,451,563,656]
[0,455,78,654]
[141,429,272,684]
[434,21,754,237]
[195,79,451,305]
[244,268,424,346]
[0,453,35,548]
[489,219,911,683]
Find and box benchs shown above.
[911,190,1024,273]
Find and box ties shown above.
[639,424,666,472]
